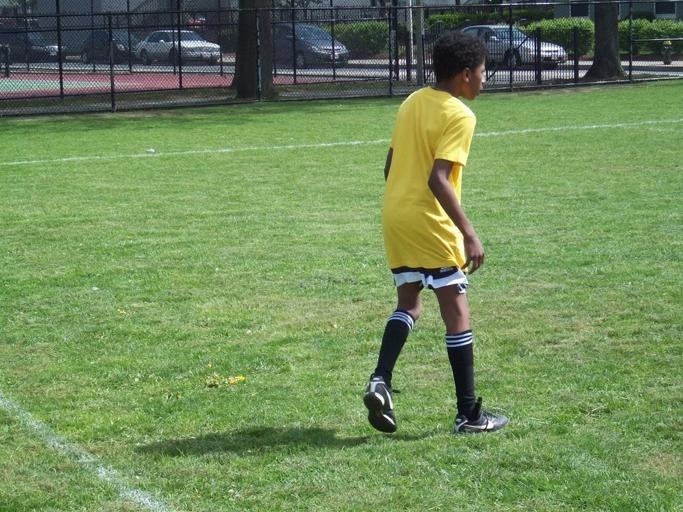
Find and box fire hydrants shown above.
[660,39,676,65]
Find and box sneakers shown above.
[454,410,509,435]
[363,372,397,432]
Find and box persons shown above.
[360,31,508,434]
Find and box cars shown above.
[136,30,221,66]
[270,24,350,70]
[80,30,142,67]
[4,27,66,62]
[456,24,569,70]
